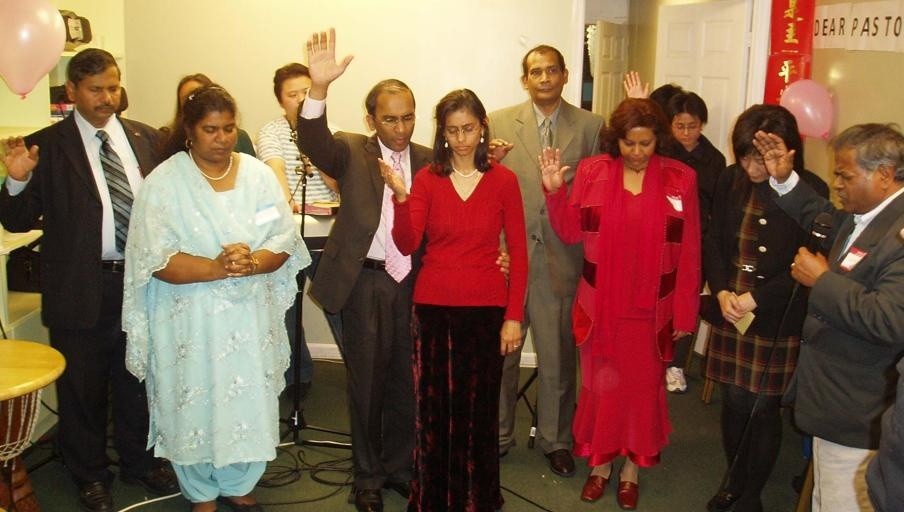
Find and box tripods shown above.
[272,188,352,454]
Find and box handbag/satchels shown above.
[6,234,42,293]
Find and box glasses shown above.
[444,124,481,137]
[374,114,416,127]
[670,124,700,132]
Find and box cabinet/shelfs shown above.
[0,130,65,336]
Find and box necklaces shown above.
[187,150,234,181]
[450,165,478,180]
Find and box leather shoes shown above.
[581,463,614,501]
[119,457,181,495]
[544,449,576,477]
[617,463,639,510]
[387,474,411,499]
[79,481,113,512]
[216,496,263,512]
[355,488,383,512]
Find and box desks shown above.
[1,340,67,512]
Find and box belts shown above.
[362,257,385,272]
[102,261,125,275]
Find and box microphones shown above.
[791,213,834,255]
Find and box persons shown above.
[747,121,903,512]
[619,68,727,394]
[536,96,703,509]
[123,83,315,512]
[374,87,530,511]
[0,46,180,511]
[295,26,444,512]
[862,352,902,512]
[254,61,346,399]
[648,83,684,112]
[162,74,258,160]
[483,42,609,477]
[700,102,831,512]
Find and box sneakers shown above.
[665,367,687,393]
[706,489,741,512]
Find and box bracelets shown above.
[250,255,260,274]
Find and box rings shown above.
[513,345,519,348]
[790,263,796,267]
[232,260,235,265]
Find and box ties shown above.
[384,151,412,284]
[95,130,134,254]
[542,117,553,147]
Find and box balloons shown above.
[0,0,66,100]
[777,79,835,149]
